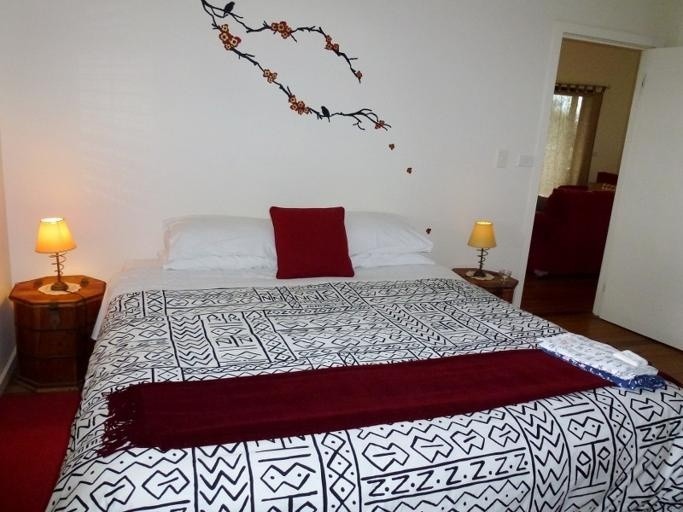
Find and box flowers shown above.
[197,1,400,156]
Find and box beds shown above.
[47,203,681,512]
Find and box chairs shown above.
[526,168,618,276]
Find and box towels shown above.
[535,329,666,390]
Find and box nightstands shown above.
[454,264,522,306]
[9,277,108,391]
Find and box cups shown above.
[498,269,512,285]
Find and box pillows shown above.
[160,214,269,257]
[162,254,273,278]
[346,252,436,270]
[340,210,433,254]
[267,205,355,279]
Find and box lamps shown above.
[35,217,80,293]
[464,220,496,277]
